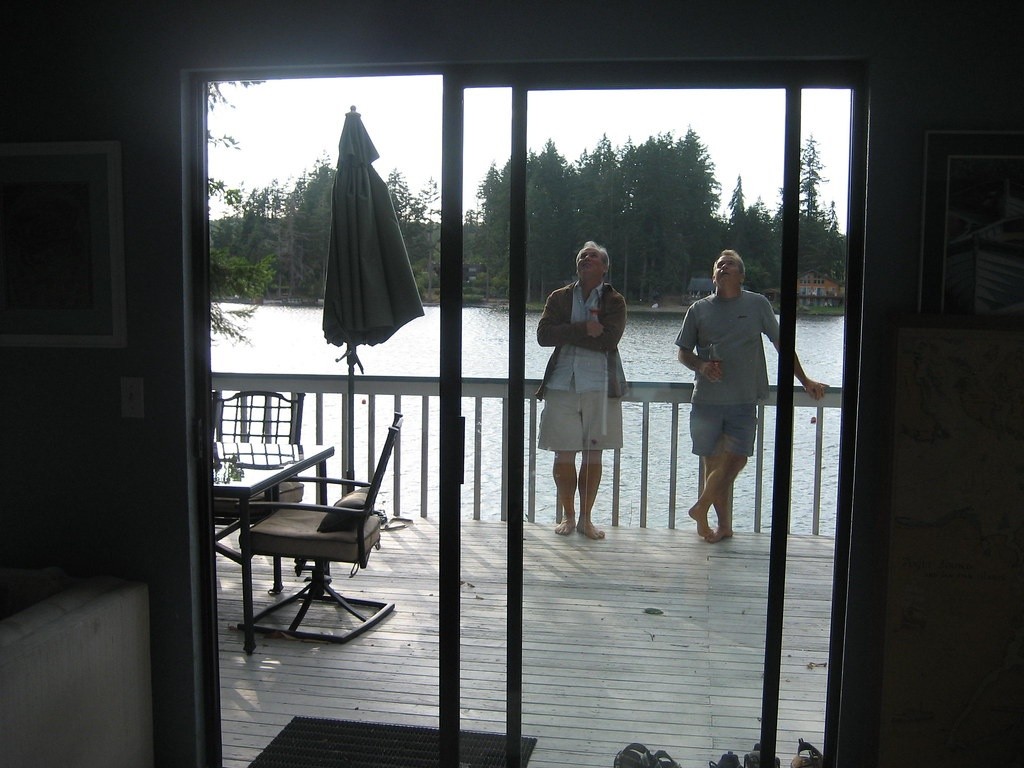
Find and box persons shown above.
[535,241,628,539]
[675,249,826,543]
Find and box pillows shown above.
[317,487,373,532]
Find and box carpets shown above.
[246,715,538,768]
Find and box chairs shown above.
[213,389,305,593]
[237,412,405,645]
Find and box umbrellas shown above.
[322,114,424,493]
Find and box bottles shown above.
[709,343,722,383]
[588,306,600,337]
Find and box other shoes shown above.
[615,743,653,768]
[790,738,823,768]
[651,750,682,768]
[707,751,743,768]
[743,743,780,768]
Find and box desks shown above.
[212,441,336,653]
